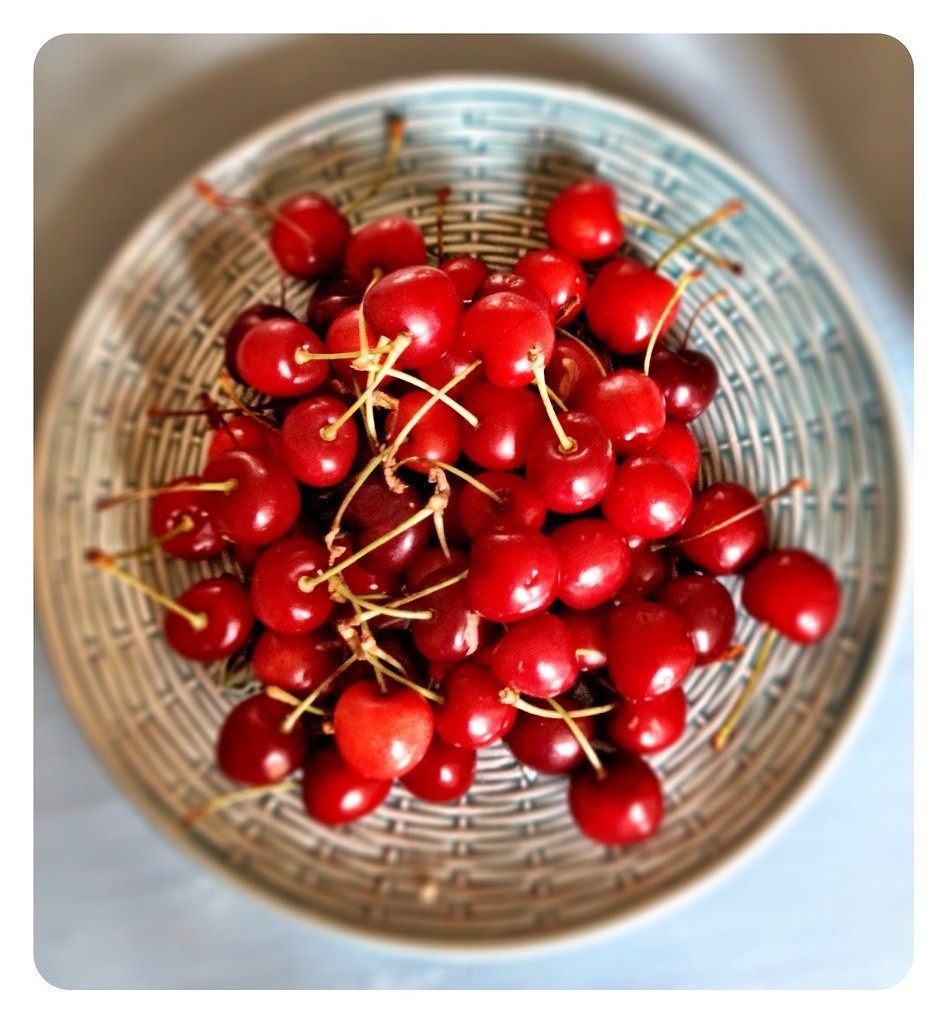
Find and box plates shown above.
[33,76,909,948]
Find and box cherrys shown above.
[88,112,841,847]
